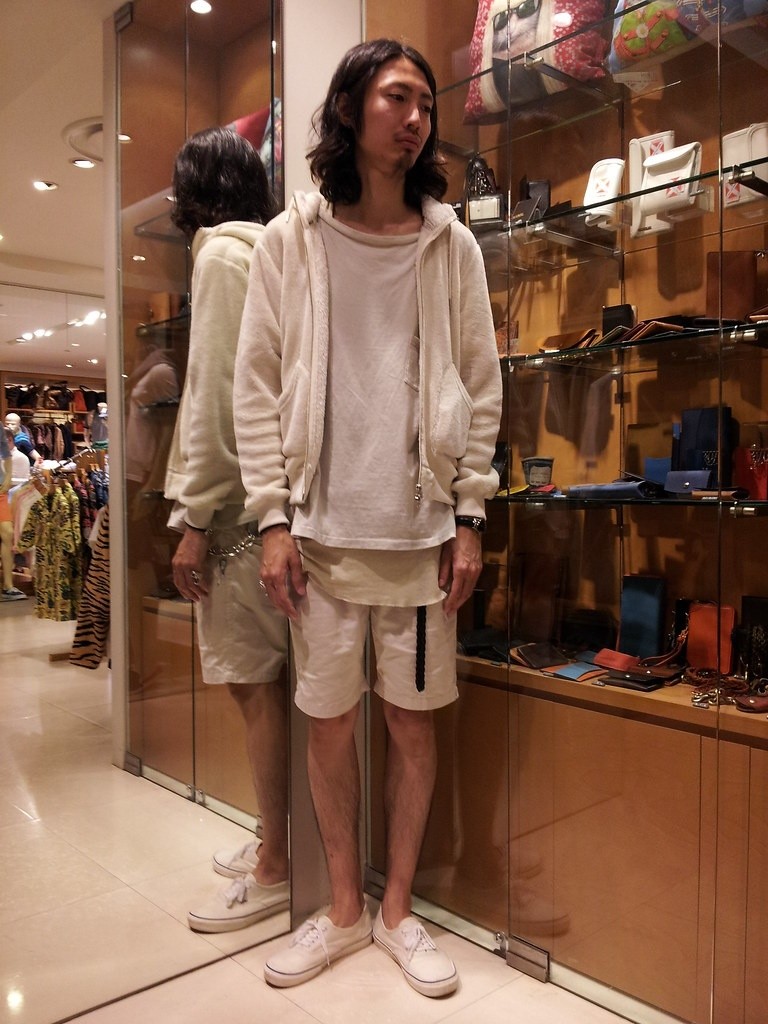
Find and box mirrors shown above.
[0,0,296,1024]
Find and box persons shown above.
[236,39,503,997]
[151,128,293,933]
[0,427,30,485]
[0,422,26,599]
[83,402,108,453]
[5,413,43,468]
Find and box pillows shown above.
[459,0,768,127]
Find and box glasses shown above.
[493,0,543,30]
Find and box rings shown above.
[258,580,267,591]
[191,570,200,585]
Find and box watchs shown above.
[455,515,486,535]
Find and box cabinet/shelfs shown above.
[103,0,286,836]
[0,371,107,454]
[356,0,768,1024]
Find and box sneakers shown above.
[186,872,290,933]
[263,901,372,987]
[211,841,263,880]
[371,903,459,998]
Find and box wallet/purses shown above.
[663,469,713,495]
[690,486,750,501]
[568,479,655,500]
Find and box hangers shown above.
[29,448,100,496]
